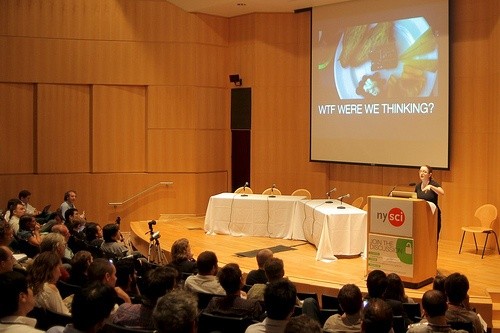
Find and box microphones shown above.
[338,194,350,200]
[243,182,249,187]
[271,184,276,188]
[326,188,336,194]
[388,183,416,196]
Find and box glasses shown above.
[27,284,33,290]
[419,170,429,173]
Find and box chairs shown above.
[12,187,500,333]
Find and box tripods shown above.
[147,225,169,264]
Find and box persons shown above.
[239,249,487,333]
[201,263,267,323]
[0,190,226,333]
[415,165,445,242]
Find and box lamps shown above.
[229,74,243,86]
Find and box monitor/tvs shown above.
[392,191,417,199]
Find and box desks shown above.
[203,192,307,241]
[292,199,368,263]
